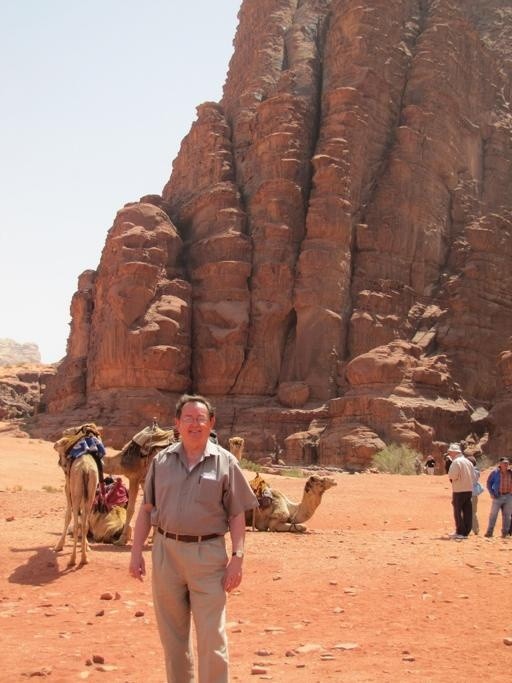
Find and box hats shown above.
[447,444,462,452]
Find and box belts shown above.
[157,526,219,542]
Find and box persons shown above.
[423,442,511,540]
[129,394,261,683]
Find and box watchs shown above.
[231,550,244,557]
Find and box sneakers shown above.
[447,532,463,538]
